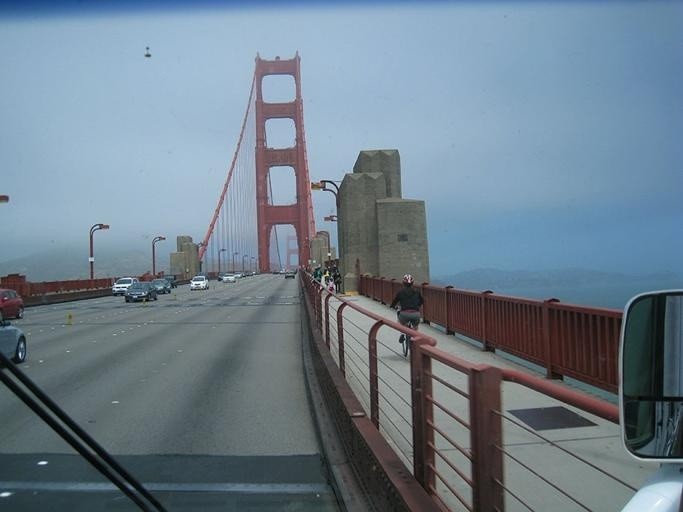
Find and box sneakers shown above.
[398,334,404,343]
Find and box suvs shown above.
[111,277,139,296]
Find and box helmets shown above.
[402,275,413,284]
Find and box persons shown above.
[311,265,342,293]
[389,274,424,343]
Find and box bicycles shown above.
[390,306,417,357]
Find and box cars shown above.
[272,267,297,280]
[0,288,25,321]
[151,274,177,294]
[124,280,157,303]
[188,275,209,291]
[0,318,26,367]
[193,270,256,283]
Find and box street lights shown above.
[322,214,337,222]
[217,248,256,272]
[151,234,165,275]
[0,193,9,204]
[89,223,109,278]
[315,231,331,261]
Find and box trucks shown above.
[625,296,683,458]
